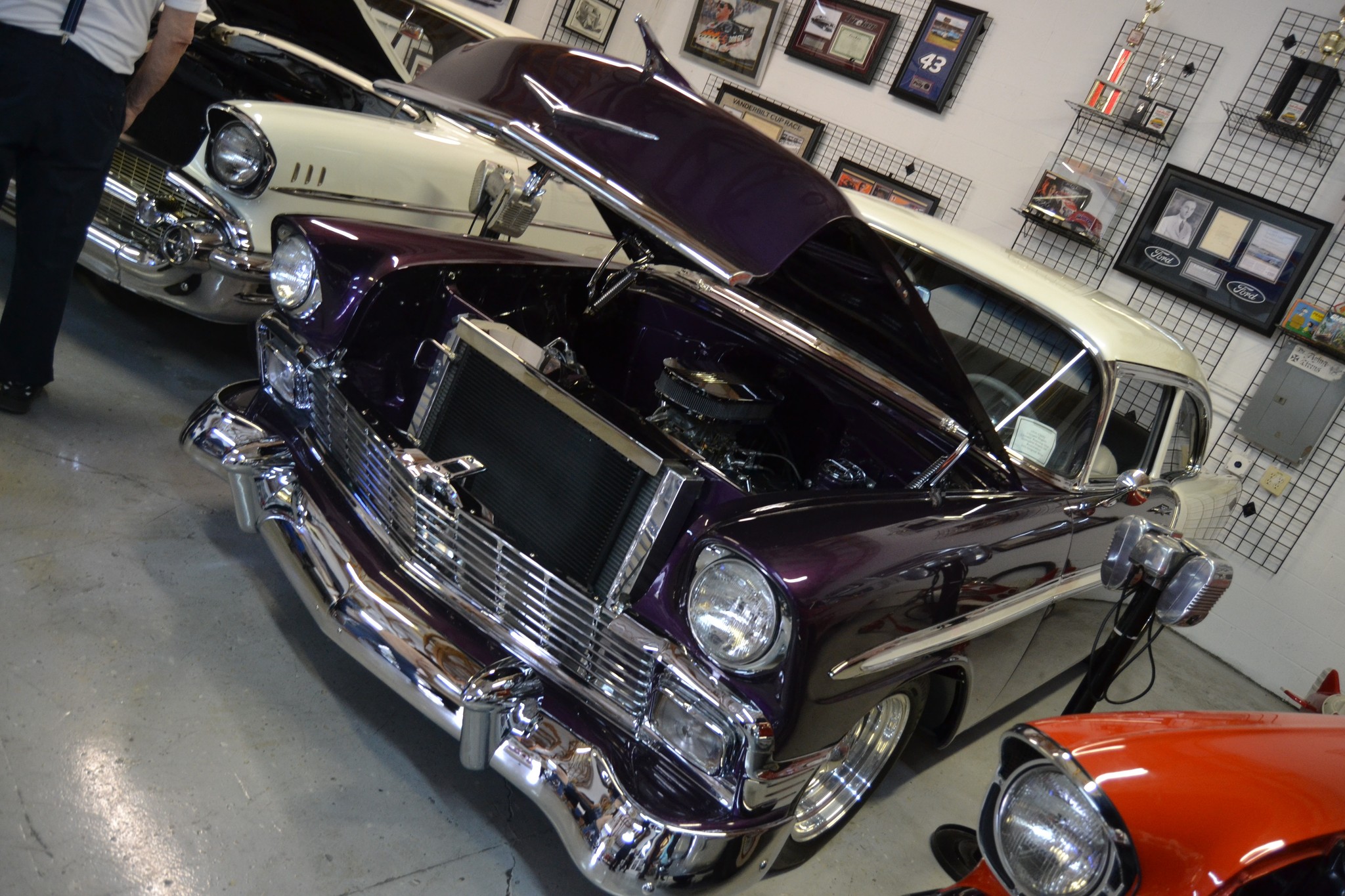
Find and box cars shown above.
[0,0,636,328]
[179,16,1216,895]
[906,711,1344,896]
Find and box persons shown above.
[1035,180,1062,213]
[1301,321,1314,333]
[1309,325,1318,337]
[1156,197,1196,247]
[695,0,739,56]
[0,2,206,417]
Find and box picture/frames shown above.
[830,156,941,217]
[888,0,990,114]
[1110,162,1336,340]
[561,0,621,45]
[714,83,827,162]
[679,0,785,84]
[785,0,901,86]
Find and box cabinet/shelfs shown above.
[1064,99,1335,168]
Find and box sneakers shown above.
[0,379,42,414]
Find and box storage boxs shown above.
[1025,151,1130,245]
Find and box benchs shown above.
[941,327,1148,475]
